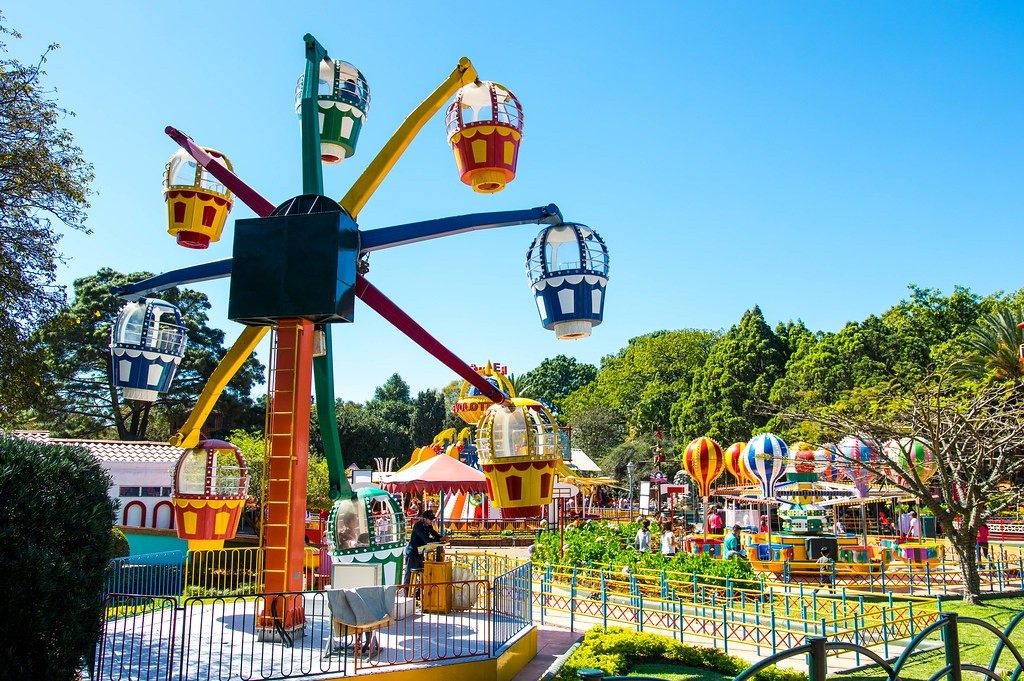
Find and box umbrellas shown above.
[382,453,487,539]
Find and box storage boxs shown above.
[330,563,382,589]
[394,597,417,621]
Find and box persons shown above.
[606,493,637,508]
[566,495,591,509]
[660,522,679,554]
[815,547,835,593]
[897,508,920,537]
[407,497,418,516]
[635,519,652,552]
[976,526,996,570]
[702,505,723,534]
[573,516,579,525]
[340,79,358,101]
[404,511,448,600]
[879,512,895,535]
[376,514,389,542]
[724,525,740,560]
[341,513,369,549]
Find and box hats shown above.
[908,511,915,515]
[423,510,436,520]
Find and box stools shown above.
[408,568,424,607]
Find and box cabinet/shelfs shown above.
[423,560,453,615]
